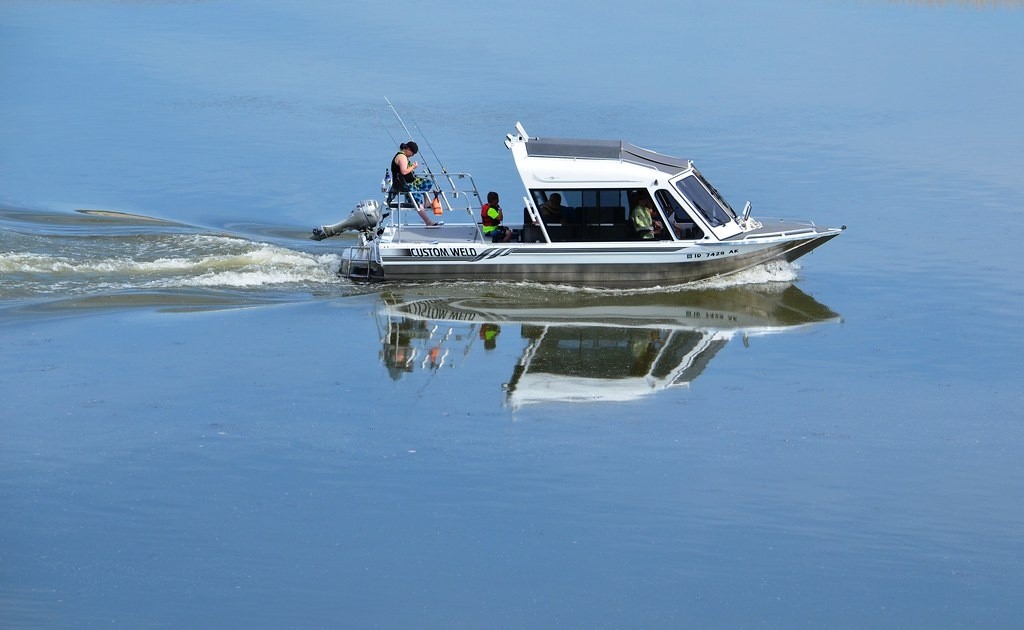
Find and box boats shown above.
[311,96,846,286]
[368,285,844,412]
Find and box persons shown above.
[481,192,511,242]
[538,193,565,223]
[631,191,662,239]
[391,142,438,226]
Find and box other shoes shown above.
[426,221,444,229]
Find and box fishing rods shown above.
[374,115,434,207]
[382,94,453,211]
[410,115,460,199]
